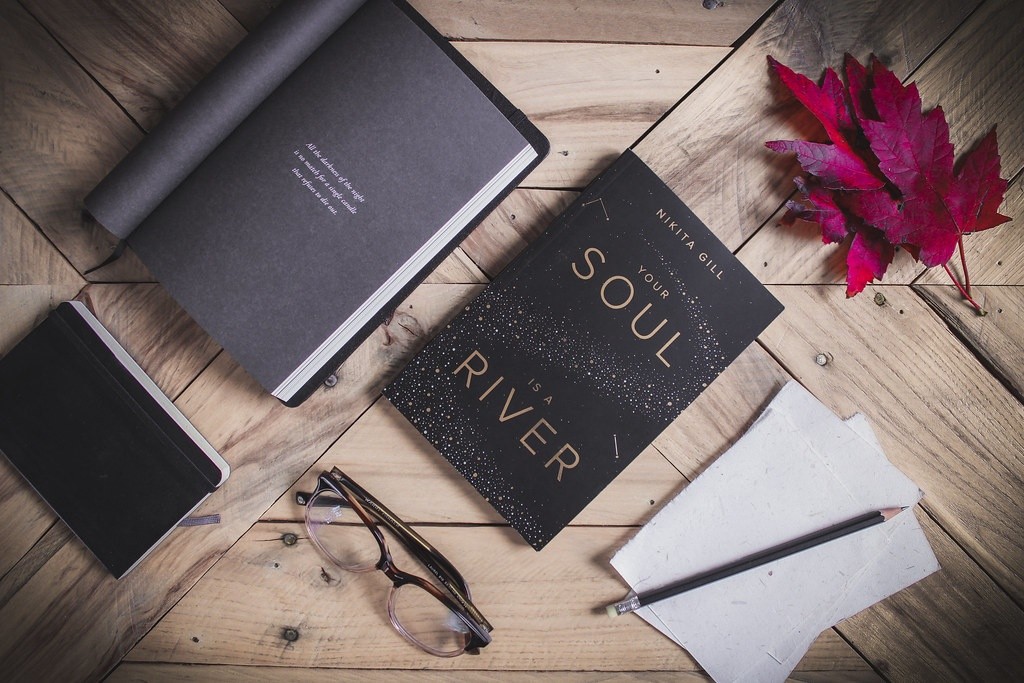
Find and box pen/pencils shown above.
[606,505,909,617]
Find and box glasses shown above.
[295,467,493,657]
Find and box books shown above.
[0,298,231,579]
[82,2,554,412]
[380,149,787,552]
